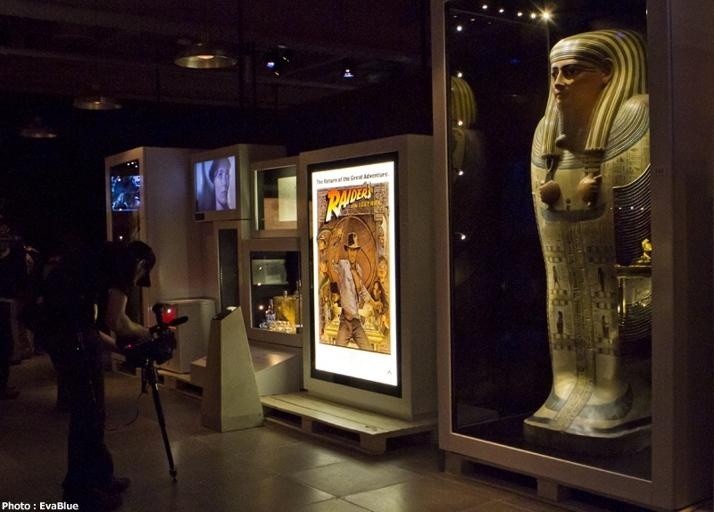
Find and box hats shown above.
[344,233,360,251]
[129,241,156,286]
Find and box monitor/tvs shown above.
[188,144,253,220]
[109,173,145,214]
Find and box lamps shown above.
[74,96,122,110]
[18,123,57,139]
[264,48,294,78]
[342,68,353,78]
[174,47,239,68]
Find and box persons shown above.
[206,158,233,209]
[38,240,154,512]
[319,229,389,352]
[0,213,25,401]
[526,28,651,439]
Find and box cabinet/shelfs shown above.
[238,156,303,348]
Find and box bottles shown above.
[269,319,296,334]
[263,305,274,328]
